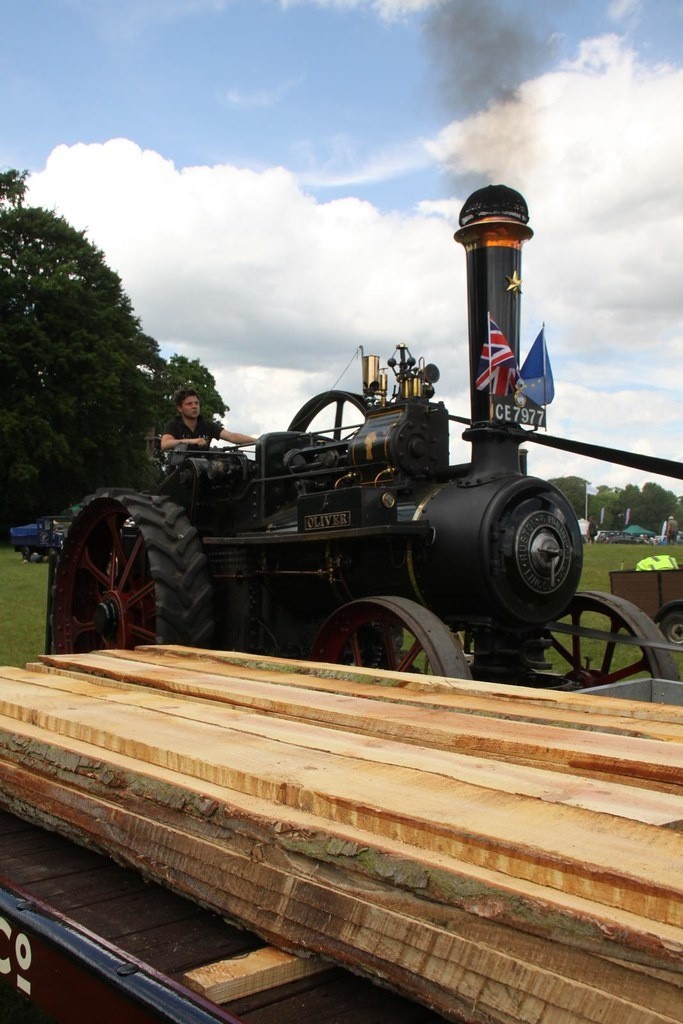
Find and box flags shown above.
[587,484,599,496]
[473,309,554,407]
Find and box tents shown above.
[623,524,656,545]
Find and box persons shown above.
[587,515,597,542]
[667,516,678,545]
[160,386,258,456]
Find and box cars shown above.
[598,530,642,544]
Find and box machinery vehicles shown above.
[46,185,683,691]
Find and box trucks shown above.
[9,514,72,561]
